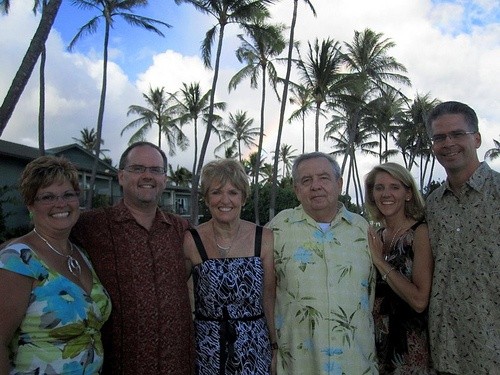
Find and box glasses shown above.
[32,190,83,204]
[430,130,477,145]
[122,165,165,175]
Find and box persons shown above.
[68,142,194,375]
[0,155,113,375]
[365,163,434,375]
[184,159,279,375]
[423,102,500,375]
[265,153,379,375]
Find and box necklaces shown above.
[389,227,401,250]
[33,228,81,278]
[212,221,240,251]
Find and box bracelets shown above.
[270,343,278,350]
[382,268,395,282]
